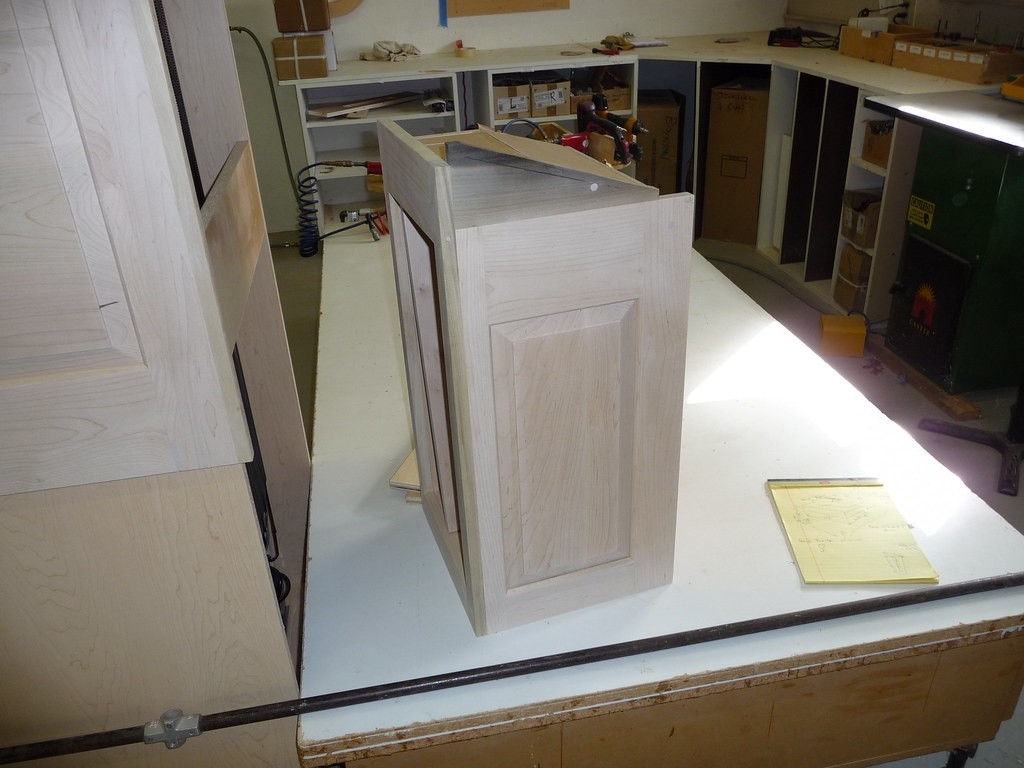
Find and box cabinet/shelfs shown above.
[283,30,1024,768]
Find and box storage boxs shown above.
[862,117,893,167]
[636,89,682,193]
[493,76,531,120]
[835,27,936,65]
[283,29,339,71]
[892,38,1024,86]
[569,71,631,113]
[272,33,328,80]
[526,71,571,117]
[849,17,890,32]
[834,274,867,314]
[842,186,883,247]
[273,0,331,32]
[839,243,872,286]
[701,77,770,243]
